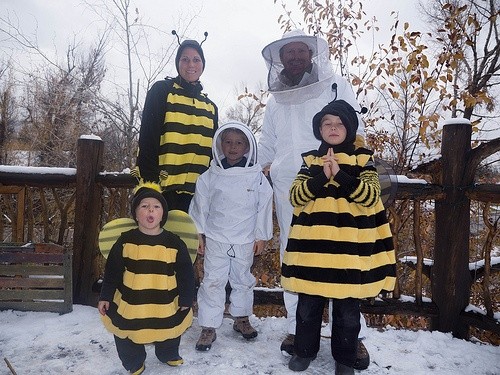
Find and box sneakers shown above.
[232,316,258,339]
[196,327,217,350]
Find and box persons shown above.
[191,120,272,349]
[281,100,397,375]
[138,39,219,215]
[97,186,195,375]
[256,29,371,371]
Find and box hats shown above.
[130,186,168,227]
[261,30,328,64]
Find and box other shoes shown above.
[288,355,316,372]
[130,363,145,375]
[352,341,370,370]
[280,333,296,358]
[166,353,183,367]
[335,363,355,375]
